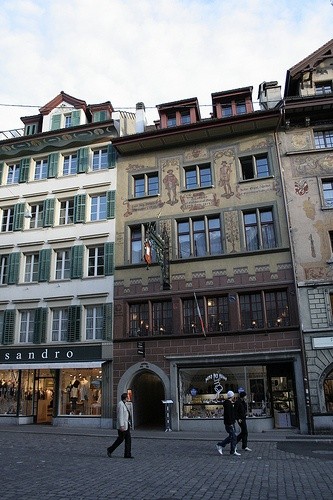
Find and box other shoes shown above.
[124,455,134,458]
[245,446,253,452]
[234,448,242,455]
[107,447,111,457]
[216,444,223,455]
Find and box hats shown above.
[227,391,234,398]
[240,392,246,398]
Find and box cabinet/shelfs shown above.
[183,392,235,405]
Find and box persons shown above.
[107,393,135,458]
[39,387,53,405]
[215,392,241,456]
[69,374,81,415]
[78,378,89,415]
[234,392,252,451]
[0,378,7,399]
